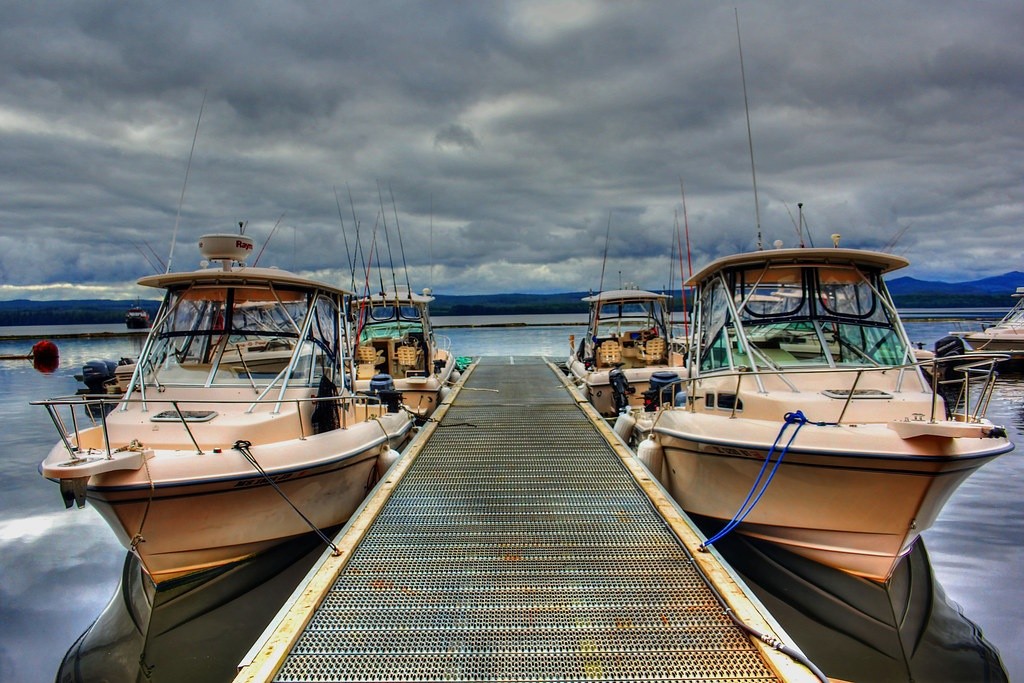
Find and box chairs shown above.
[595,340,623,367]
[397,345,422,369]
[356,345,385,366]
[636,338,665,367]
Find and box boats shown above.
[618,10,1016,577]
[949,286,1024,367]
[124,306,149,329]
[29,101,417,591]
[698,526,1010,683]
[565,211,699,418]
[53,526,340,683]
[668,288,968,394]
[71,175,457,428]
